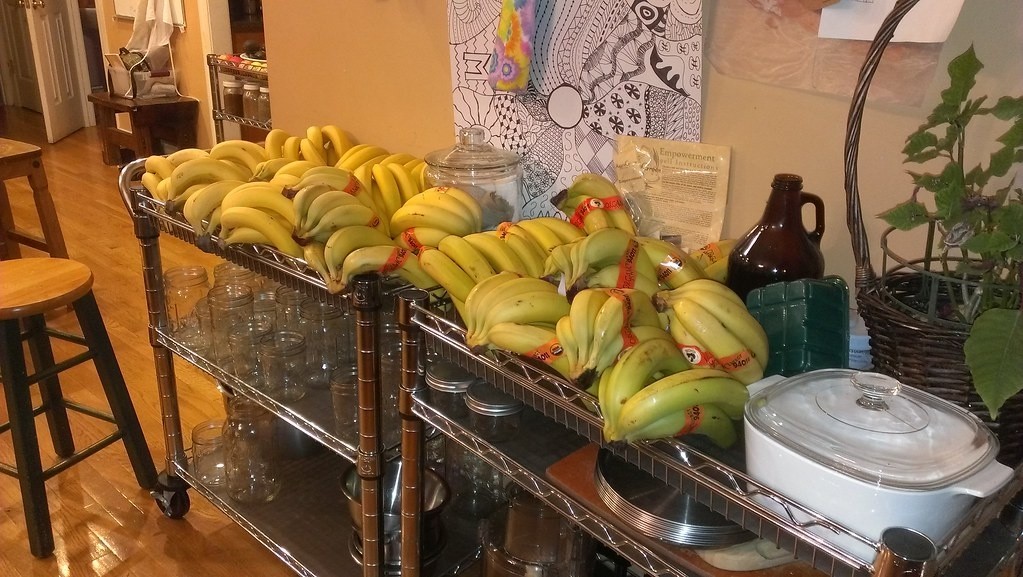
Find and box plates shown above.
[594,446,759,548]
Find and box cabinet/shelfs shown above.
[397,288,1023,577]
[207,54,272,143]
[118,148,418,577]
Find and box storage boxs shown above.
[107,64,182,100]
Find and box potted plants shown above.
[880,43,1023,417]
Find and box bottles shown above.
[243,84,260,122]
[222,80,242,120]
[421,128,522,233]
[258,87,271,125]
[725,173,825,298]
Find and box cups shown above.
[164,256,525,517]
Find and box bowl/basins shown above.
[339,461,459,576]
[741,365,1013,563]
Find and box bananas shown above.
[418,171,770,450]
[141,124,486,310]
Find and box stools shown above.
[0,257,159,561]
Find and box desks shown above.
[0,137,75,313]
[88,93,199,167]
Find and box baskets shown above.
[845,0,1023,460]
